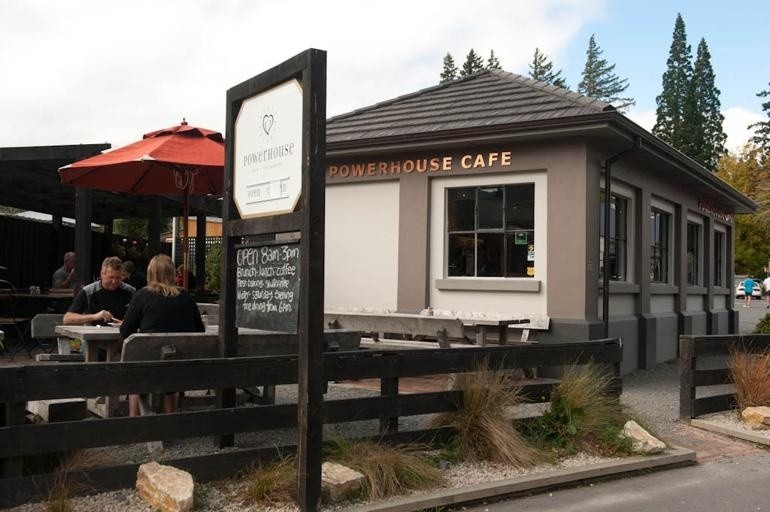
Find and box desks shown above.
[0,294,74,352]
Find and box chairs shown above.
[0,280,46,360]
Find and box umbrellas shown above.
[57,117,226,291]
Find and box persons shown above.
[119,253,206,416]
[763,272,770,308]
[176,265,196,289]
[121,261,148,291]
[52,251,77,289]
[63,256,137,405]
[742,275,755,308]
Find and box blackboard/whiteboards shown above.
[235,238,301,334]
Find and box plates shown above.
[107,323,122,328]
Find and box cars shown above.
[736,281,761,300]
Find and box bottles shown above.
[202,314,208,329]
[30,286,40,296]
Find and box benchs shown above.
[31,303,551,421]
